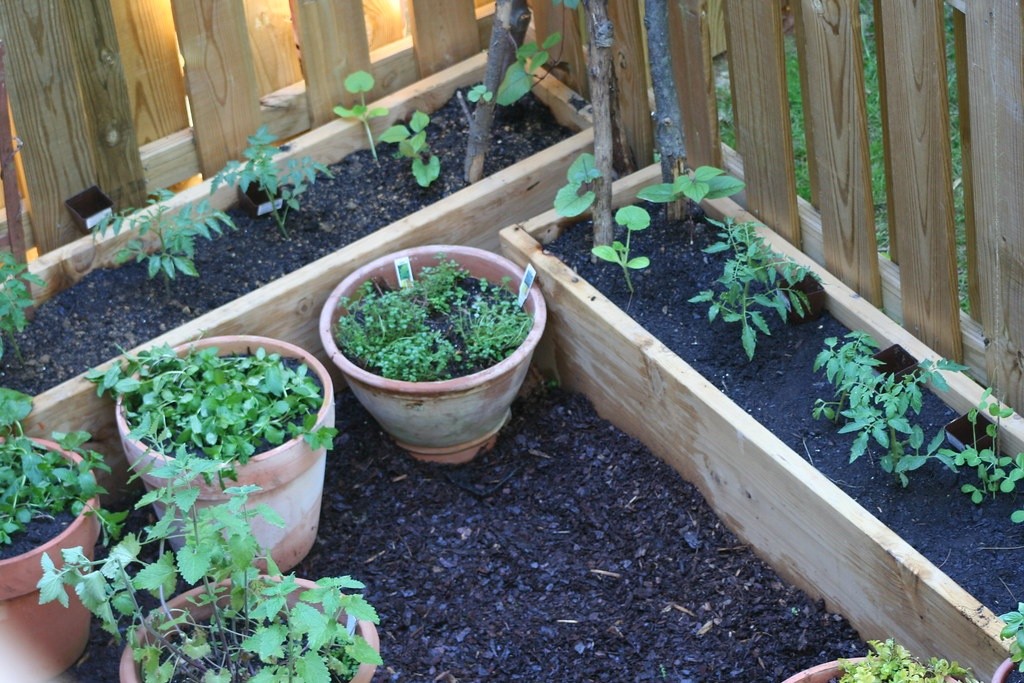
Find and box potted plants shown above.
[1,25,609,509]
[36,411,383,683]
[0,386,130,683]
[318,243,546,466]
[779,637,964,683]
[498,157,1023,682]
[87,334,340,578]
